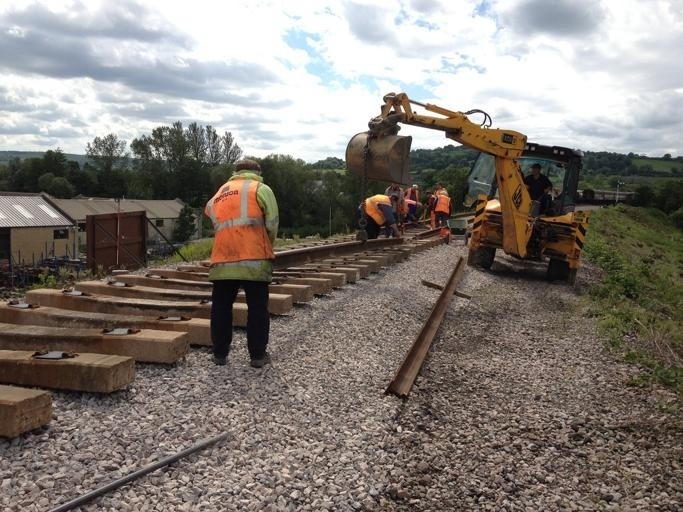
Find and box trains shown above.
[578,189,635,204]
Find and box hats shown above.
[530,164,541,168]
[390,181,443,203]
[235,158,262,174]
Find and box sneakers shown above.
[211,355,227,366]
[251,352,271,368]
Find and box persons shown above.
[525,162,553,206]
[358,182,453,239]
[205,160,280,367]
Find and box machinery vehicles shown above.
[346,92,592,284]
